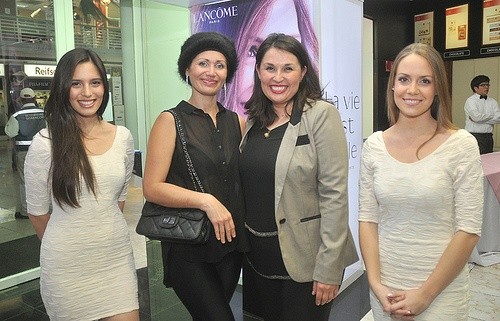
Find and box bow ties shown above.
[479,95,487,100]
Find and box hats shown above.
[20,88,35,98]
[177,31,238,86]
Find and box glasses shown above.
[479,84,490,87]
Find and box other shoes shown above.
[14,212,29,219]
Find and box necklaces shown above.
[261,108,292,138]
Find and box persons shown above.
[24,48,140,321]
[4,88,49,218]
[358,43,484,321]
[464,74,500,155]
[190,0,319,124]
[238,34,360,321]
[142,32,247,321]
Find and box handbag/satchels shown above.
[136,110,211,245]
